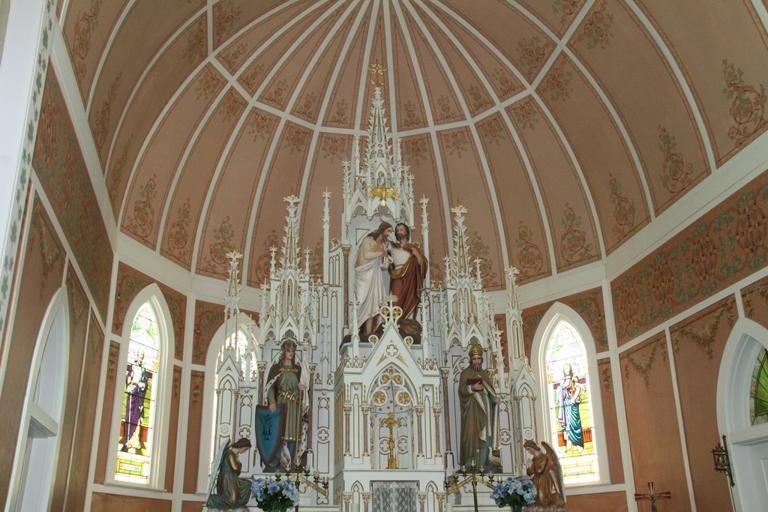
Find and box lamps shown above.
[711,433,733,485]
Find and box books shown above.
[467,379,482,386]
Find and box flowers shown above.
[249,474,299,512]
[491,473,536,510]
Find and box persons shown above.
[524,440,566,507]
[263,340,306,473]
[458,343,501,472]
[207,438,253,509]
[353,220,429,335]
[557,362,584,452]
[118,351,147,455]
